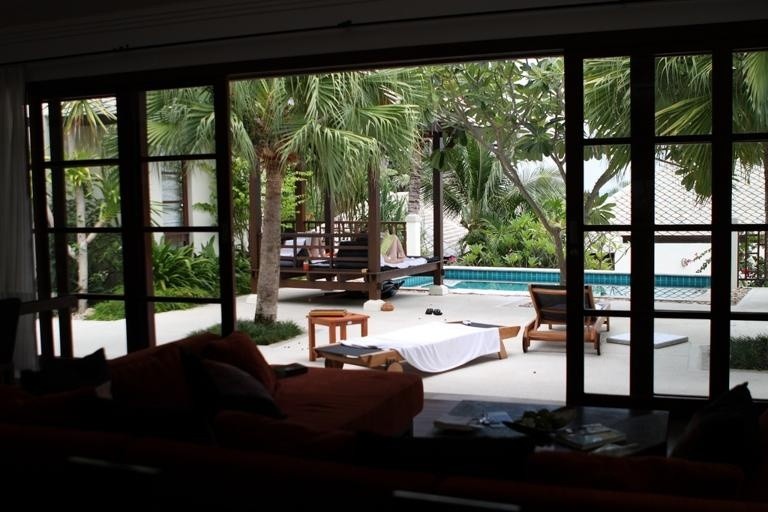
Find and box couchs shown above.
[1,417,768,511]
[101,333,424,458]
[1,332,767,512]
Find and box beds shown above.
[250,221,443,299]
[312,319,521,372]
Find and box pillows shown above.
[147,331,288,423]
[23,350,107,399]
[667,380,757,461]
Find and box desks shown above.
[0,292,78,316]
[436,399,669,474]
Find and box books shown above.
[548,421,628,453]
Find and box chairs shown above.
[522,282,611,353]
[0,297,21,386]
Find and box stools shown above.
[307,312,370,362]
